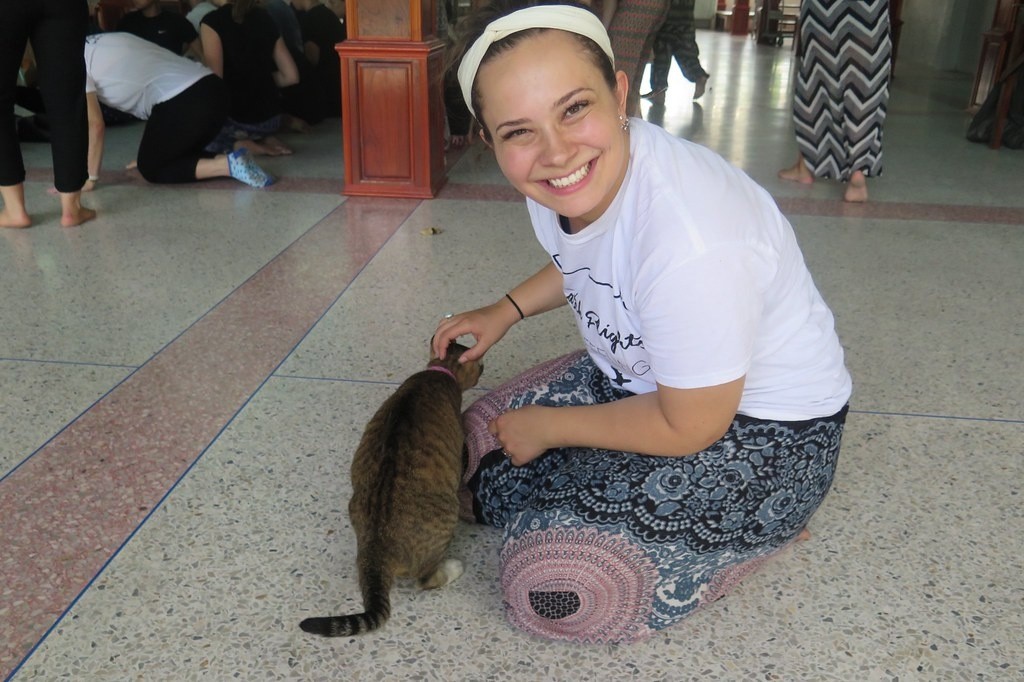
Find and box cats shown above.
[299,335,484,639]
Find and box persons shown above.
[0,0,99,229]
[45,30,275,194]
[104,0,349,157]
[778,0,893,204]
[432,6,854,647]
[639,0,709,104]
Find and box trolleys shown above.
[758,0,798,47]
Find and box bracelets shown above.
[506,293,524,320]
[88,175,100,181]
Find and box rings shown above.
[443,313,455,322]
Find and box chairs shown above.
[761,0,799,50]
